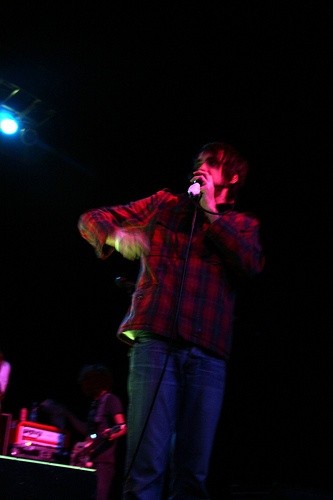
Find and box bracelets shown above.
[114,231,127,252]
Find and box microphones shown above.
[189,180,202,198]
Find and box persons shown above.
[67,365,128,500]
[77,141,264,500]
